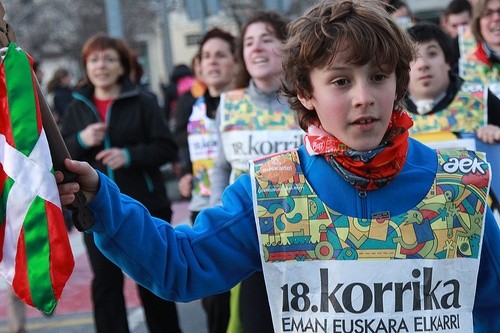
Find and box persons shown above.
[53,0,500,333]
[8,11,305,333]
[383,0,500,144]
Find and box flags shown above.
[0,46,76,316]
